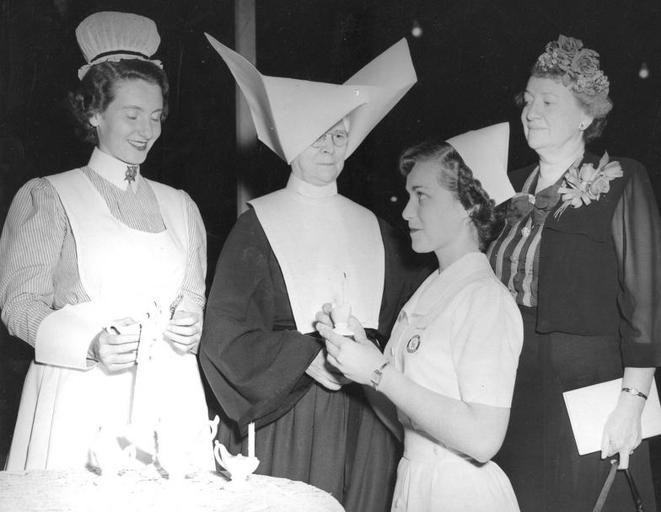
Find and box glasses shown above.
[310,129,350,148]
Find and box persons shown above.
[481,36,659,512]
[307,134,524,512]
[1,58,219,485]
[193,108,401,512]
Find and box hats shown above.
[445,121,517,207]
[75,10,166,81]
[203,31,420,166]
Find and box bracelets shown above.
[371,359,390,390]
[621,387,649,401]
